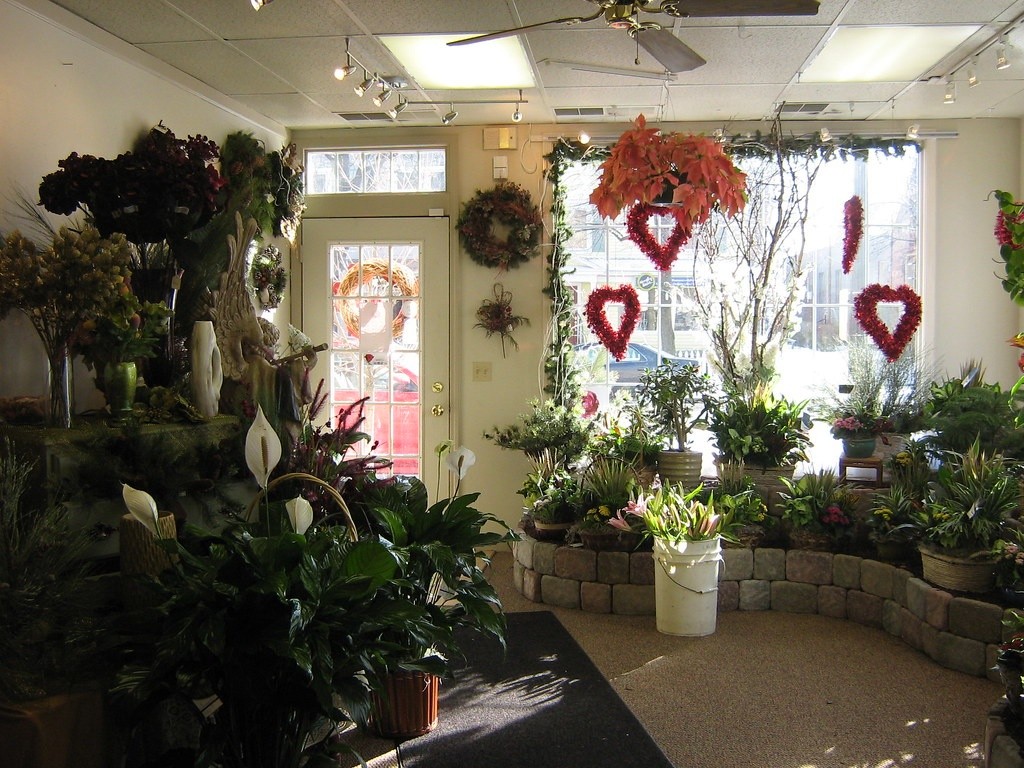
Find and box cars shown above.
[332,343,420,475]
[575,345,704,409]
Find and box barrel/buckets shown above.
[652,534,722,636]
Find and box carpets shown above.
[397,610,673,767]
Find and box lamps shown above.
[373,82,392,106]
[442,103,458,125]
[821,128,831,142]
[578,130,591,144]
[512,102,522,122]
[714,129,725,143]
[909,123,919,137]
[334,53,357,81]
[354,70,374,96]
[250,0,273,9]
[388,94,407,119]
[968,55,981,89]
[995,36,1012,69]
[944,74,956,104]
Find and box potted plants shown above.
[629,355,741,487]
[707,391,826,512]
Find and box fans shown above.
[445,0,819,73]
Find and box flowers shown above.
[0,112,1024,768]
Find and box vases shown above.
[121,512,177,578]
[358,648,446,738]
[917,547,996,594]
[43,336,73,429]
[843,439,875,458]
[647,171,686,206]
[579,528,639,552]
[534,519,571,540]
[103,361,137,427]
[131,267,185,382]
[789,527,830,553]
[874,540,919,565]
[191,321,222,418]
[876,433,910,486]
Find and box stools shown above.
[839,451,884,486]
